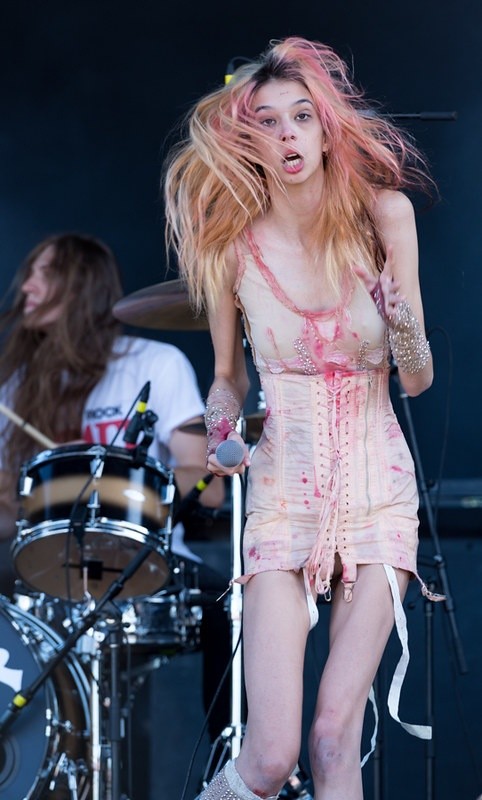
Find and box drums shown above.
[1,595,101,800]
[9,442,180,602]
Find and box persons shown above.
[165,36,434,800]
[0,232,318,800]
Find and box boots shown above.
[195,758,282,800]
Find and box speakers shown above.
[145,653,217,800]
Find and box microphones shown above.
[215,439,245,467]
[225,61,235,86]
[179,473,215,510]
[122,381,150,445]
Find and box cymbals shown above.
[111,277,210,331]
[178,411,265,443]
